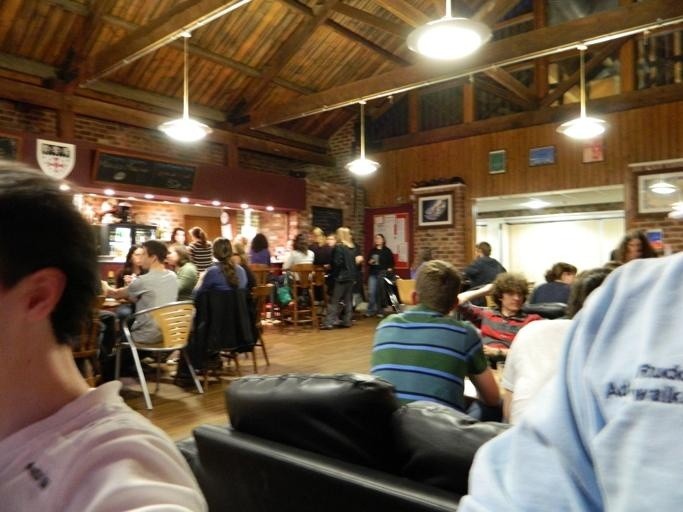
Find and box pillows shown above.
[221,372,400,473]
[393,400,514,493]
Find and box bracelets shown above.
[494,346,504,357]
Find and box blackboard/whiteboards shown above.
[92,149,196,193]
[311,206,343,236]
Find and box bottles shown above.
[106,269,116,287]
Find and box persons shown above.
[249,233,271,284]
[0,164,208,512]
[455,250,683,512]
[457,240,507,306]
[500,266,616,429]
[115,244,148,304]
[95,239,179,389]
[370,259,504,423]
[455,272,544,372]
[72,277,116,385]
[361,233,394,317]
[186,226,215,280]
[610,231,658,263]
[528,261,577,305]
[233,235,248,260]
[166,228,190,246]
[165,242,199,301]
[282,227,363,330]
[191,236,249,376]
[232,253,257,292]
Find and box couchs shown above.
[520,302,567,319]
[173,423,511,511]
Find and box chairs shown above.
[70,262,333,411]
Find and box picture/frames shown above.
[528,144,556,167]
[634,167,683,217]
[581,144,605,164]
[416,192,454,230]
[488,148,506,174]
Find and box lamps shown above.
[407,0,492,60]
[555,46,610,140]
[157,33,213,142]
[648,177,678,193]
[346,102,382,176]
